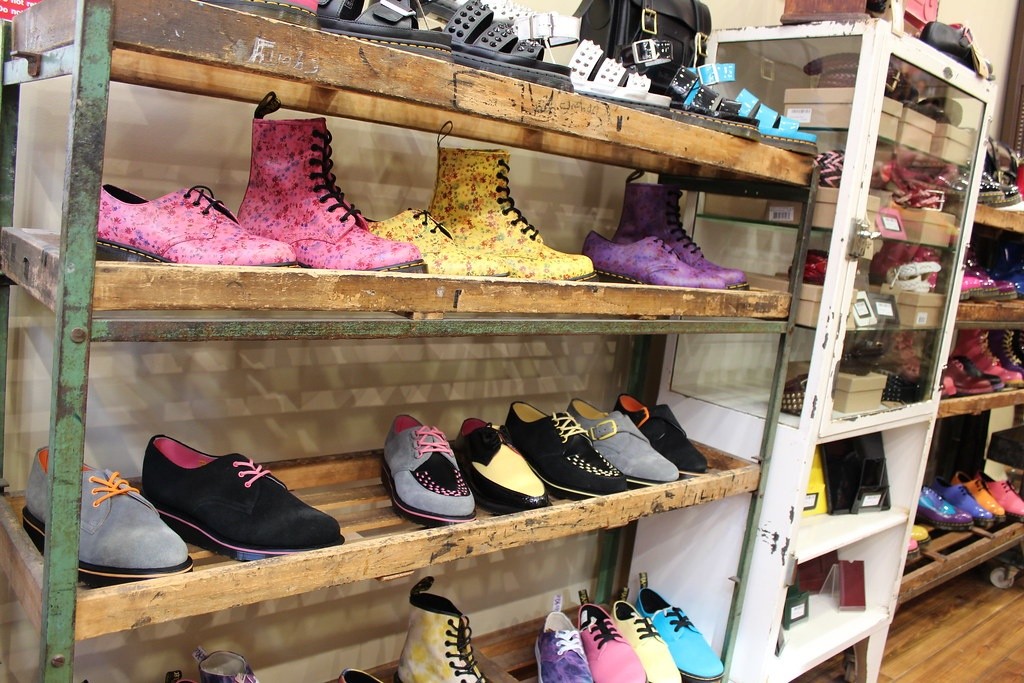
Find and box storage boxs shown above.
[834,370,887,414]
[811,186,880,235]
[870,284,946,329]
[893,207,955,248]
[929,123,972,166]
[745,271,858,332]
[898,106,937,153]
[780,0,870,25]
[782,87,904,139]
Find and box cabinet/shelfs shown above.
[897,203,1024,605]
[623,18,997,683]
[1,0,820,683]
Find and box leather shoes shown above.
[906,472,1024,554]
[869,159,1024,301]
[362,210,508,277]
[581,229,728,291]
[922,20,995,80]
[96,184,299,269]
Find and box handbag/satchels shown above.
[573,0,712,96]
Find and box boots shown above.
[613,169,751,290]
[883,329,1024,397]
[418,122,599,282]
[236,92,428,273]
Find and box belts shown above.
[803,51,912,102]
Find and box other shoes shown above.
[199,0,320,31]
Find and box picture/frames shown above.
[852,291,878,327]
[868,292,899,323]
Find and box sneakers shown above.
[20,394,708,587]
[164,575,725,683]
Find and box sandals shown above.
[512,11,672,110]
[318,0,452,53]
[682,64,818,157]
[417,0,574,93]
[619,39,761,142]
